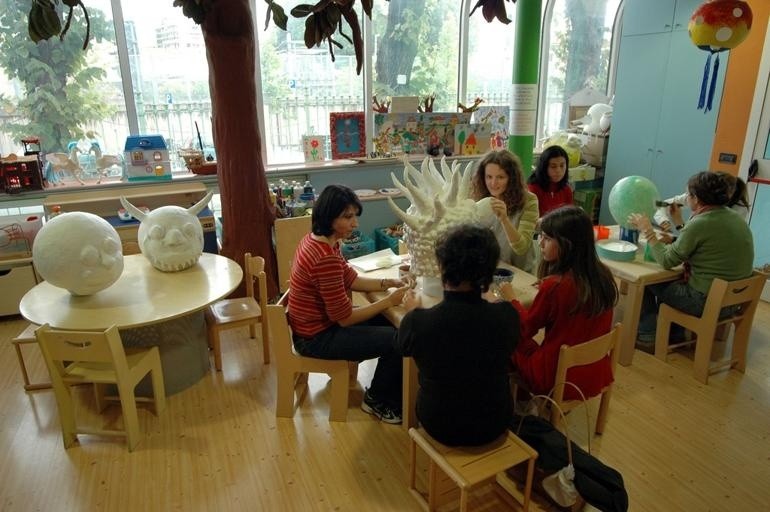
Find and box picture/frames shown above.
[330,112,367,160]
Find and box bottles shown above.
[269,178,316,208]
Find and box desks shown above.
[593,224,686,366]
[19,251,244,401]
[347,253,543,329]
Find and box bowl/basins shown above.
[595,240,638,262]
[288,203,307,216]
[398,265,417,281]
[491,268,516,284]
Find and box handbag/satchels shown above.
[506,453,591,512]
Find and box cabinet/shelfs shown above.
[599,34,729,226]
[0,266,38,317]
[621,0,714,36]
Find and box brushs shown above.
[656,201,685,207]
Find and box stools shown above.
[407,424,539,512]
[11,323,54,390]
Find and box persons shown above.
[654,170,751,245]
[499,206,614,413]
[467,151,541,273]
[526,146,575,217]
[624,171,755,347]
[290,185,418,424]
[393,226,522,454]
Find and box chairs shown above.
[204,251,270,371]
[655,269,768,384]
[266,280,359,422]
[512,322,623,435]
[34,324,168,453]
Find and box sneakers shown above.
[635,333,655,354]
[361,386,403,423]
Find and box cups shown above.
[593,226,611,239]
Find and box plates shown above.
[379,187,401,195]
[352,189,376,197]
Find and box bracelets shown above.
[676,224,685,231]
[381,276,388,292]
[671,235,676,242]
[642,228,656,243]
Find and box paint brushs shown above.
[194,121,205,163]
